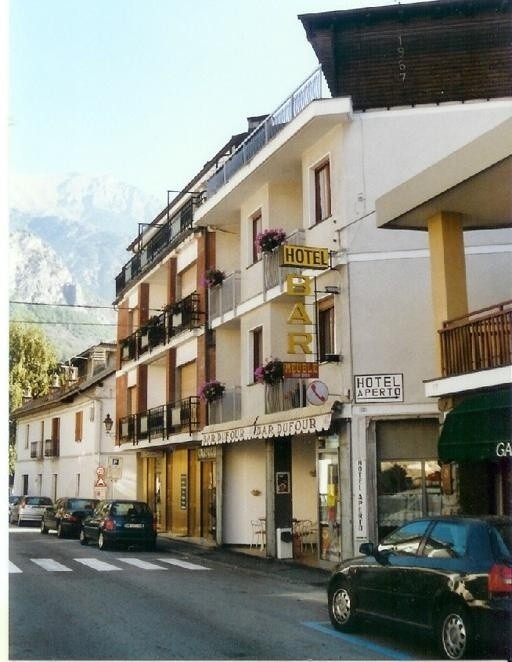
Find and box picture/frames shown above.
[276,472,291,495]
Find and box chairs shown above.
[250,517,314,554]
[128,509,138,515]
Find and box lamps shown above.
[103,413,114,436]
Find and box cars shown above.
[9,496,156,551]
[325,515,512,660]
